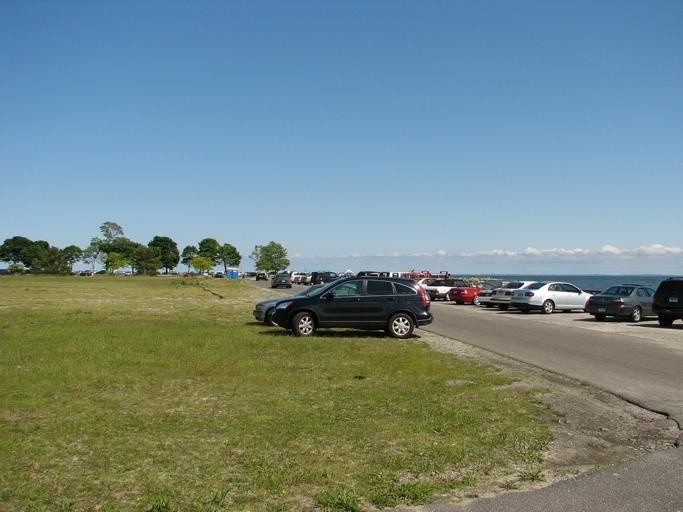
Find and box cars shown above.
[79,269,207,278]
[585,282,657,323]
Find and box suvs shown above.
[651,276,683,329]
